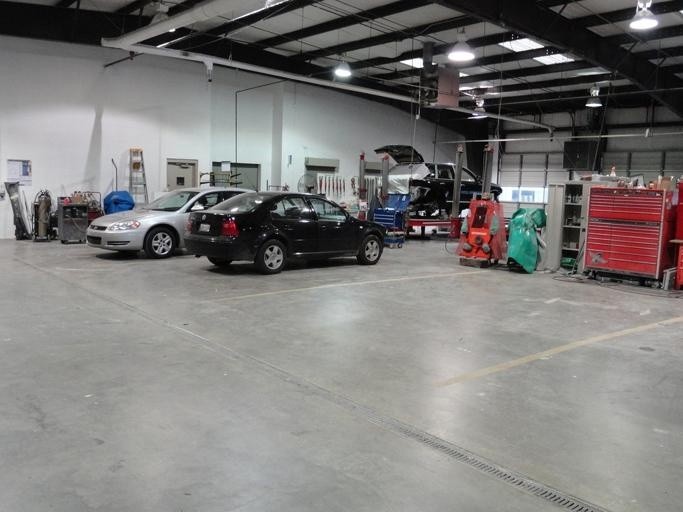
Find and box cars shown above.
[85,186,258,259]
[374,144,502,215]
[183,190,388,275]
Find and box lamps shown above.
[585,86,603,108]
[447,24,476,64]
[472,99,486,116]
[331,60,353,79]
[628,0,660,32]
[147,0,176,32]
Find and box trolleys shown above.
[374,208,406,249]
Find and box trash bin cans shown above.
[104,191,134,215]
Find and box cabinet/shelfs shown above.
[546,181,622,276]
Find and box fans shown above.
[297,173,319,195]
